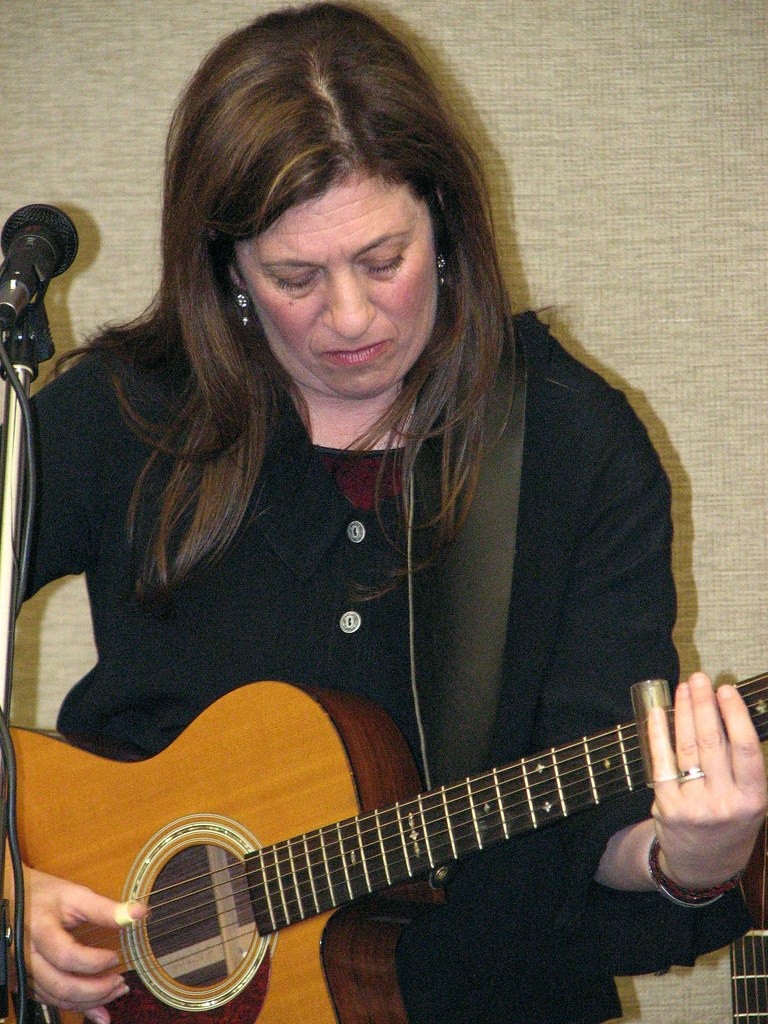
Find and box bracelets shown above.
[648,837,743,908]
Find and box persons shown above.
[1,4,768,1024]
[1,0,768,1024]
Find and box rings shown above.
[676,767,705,784]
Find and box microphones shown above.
[0,204,79,329]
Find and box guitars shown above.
[0,672,768,1024]
